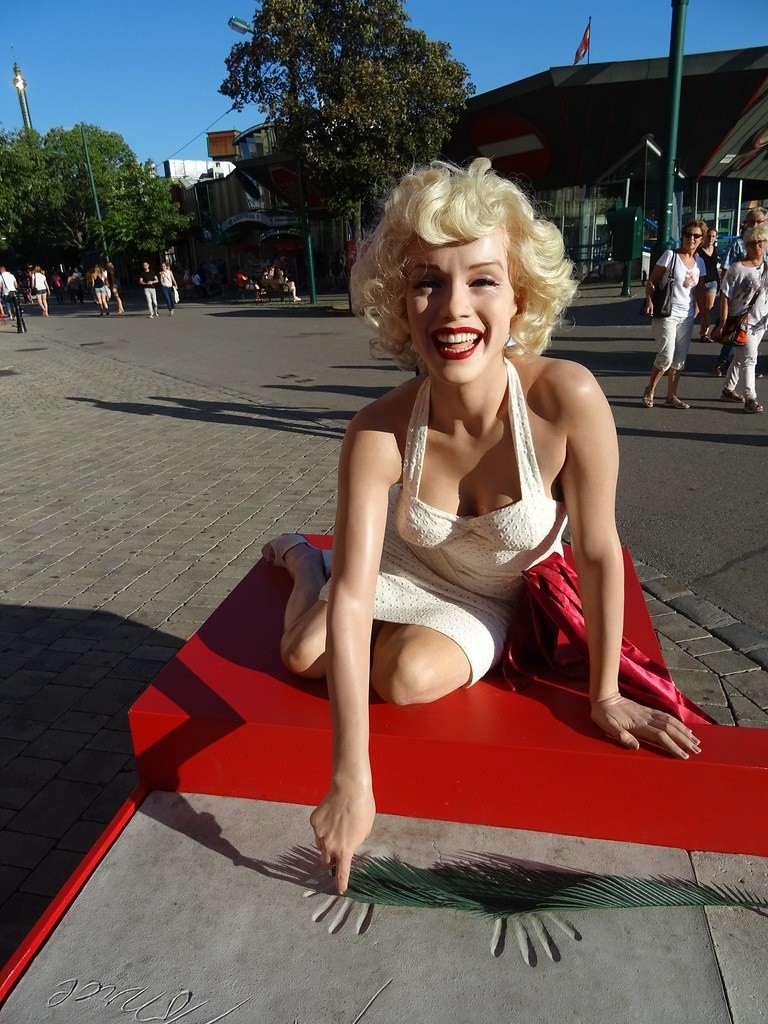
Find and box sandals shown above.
[664,394,690,408]
[744,400,763,412]
[721,389,745,402]
[643,387,654,407]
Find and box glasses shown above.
[750,240,766,245]
[685,232,702,238]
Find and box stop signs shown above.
[470,109,552,183]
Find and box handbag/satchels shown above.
[640,251,677,318]
[710,312,748,346]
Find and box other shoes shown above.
[713,360,730,378]
[292,296,302,301]
[36,299,176,319]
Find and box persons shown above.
[261,158,702,894]
[139,261,159,318]
[15,264,51,317]
[713,207,768,378]
[713,222,768,413]
[237,266,261,301]
[0,266,18,320]
[159,262,178,316]
[192,258,226,297]
[642,220,707,409]
[262,267,301,301]
[86,262,124,316]
[50,267,85,304]
[694,228,720,343]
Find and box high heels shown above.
[262,533,332,578]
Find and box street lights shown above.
[227,16,318,306]
